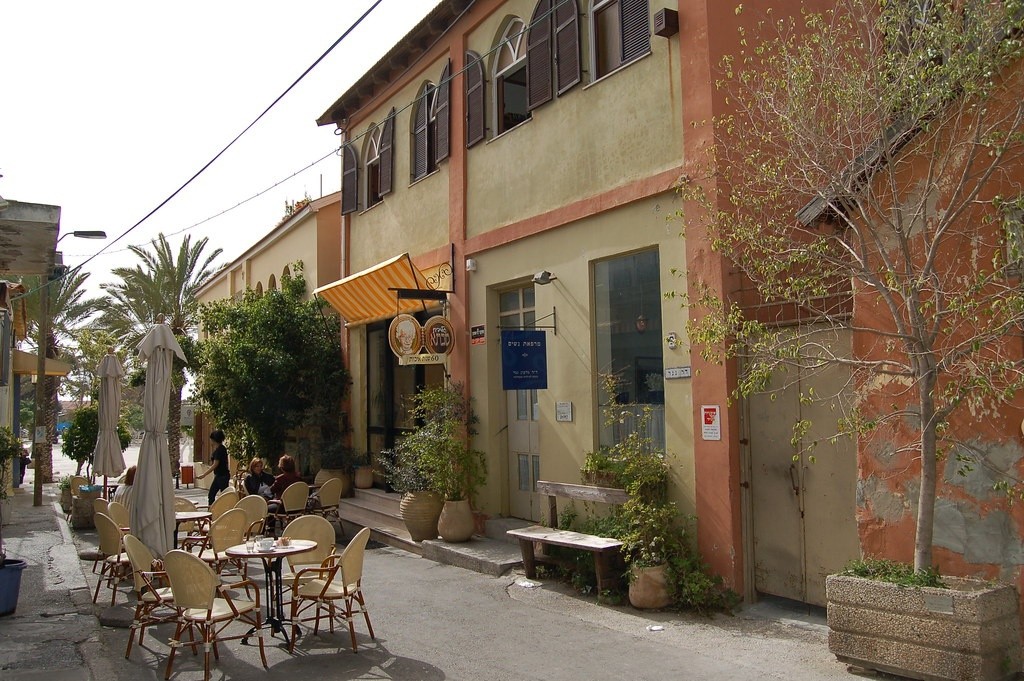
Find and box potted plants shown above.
[0,426,27,525]
[598,496,699,609]
[314,433,370,498]
[372,381,488,542]
[61,385,134,512]
[653,0,1024,681]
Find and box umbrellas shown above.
[92,354,127,500]
[130,321,188,558]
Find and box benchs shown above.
[506,480,635,603]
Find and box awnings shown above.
[313,251,442,327]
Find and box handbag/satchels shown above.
[24,458,32,465]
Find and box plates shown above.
[258,549,274,552]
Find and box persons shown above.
[114,465,137,511]
[238,455,307,538]
[196,430,230,513]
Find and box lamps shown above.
[32,374,37,384]
[531,271,558,286]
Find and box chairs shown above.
[66,476,376,681]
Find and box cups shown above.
[281,537,291,546]
[261,541,271,549]
[256,535,263,547]
[264,538,274,546]
[246,541,254,553]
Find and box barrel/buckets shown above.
[0,558,27,616]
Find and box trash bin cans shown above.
[181,466,193,484]
[20,450,29,479]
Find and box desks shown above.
[225,539,317,650]
[100,484,124,502]
[307,483,322,497]
[174,511,213,549]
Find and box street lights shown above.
[33,231,107,506]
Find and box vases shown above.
[0,559,28,617]
